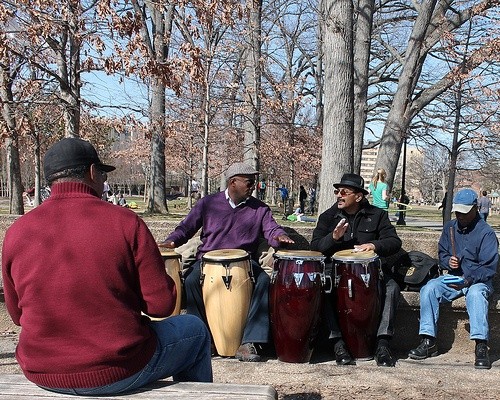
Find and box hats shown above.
[333,174,368,195]
[226,162,261,179]
[450,189,477,214]
[43,138,116,177]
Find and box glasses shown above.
[97,169,107,181]
[236,177,258,187]
[334,189,357,196]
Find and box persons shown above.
[191,178,198,192]
[101,180,110,201]
[476,191,492,222]
[299,185,307,213]
[275,184,289,204]
[156,161,296,362]
[438,192,453,226]
[2,136,214,395]
[308,187,316,216]
[257,178,262,199]
[107,192,129,208]
[408,188,499,369]
[310,172,413,366]
[368,168,392,213]
[260,178,267,200]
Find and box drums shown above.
[267,249,325,365]
[134,249,186,322]
[200,248,256,358]
[330,245,384,367]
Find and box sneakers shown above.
[235,343,260,361]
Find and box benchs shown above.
[0,373,278,400]
[397,290,500,314]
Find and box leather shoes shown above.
[475,342,491,369]
[336,346,352,364]
[376,347,394,366]
[407,337,437,358]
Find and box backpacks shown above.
[394,250,439,290]
[260,182,265,189]
